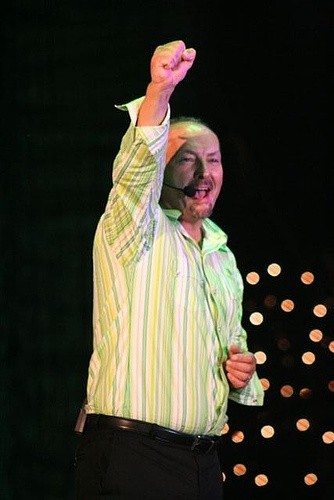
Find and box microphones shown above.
[164,183,196,197]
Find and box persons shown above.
[76,37,265,500]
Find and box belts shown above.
[85,414,230,456]
